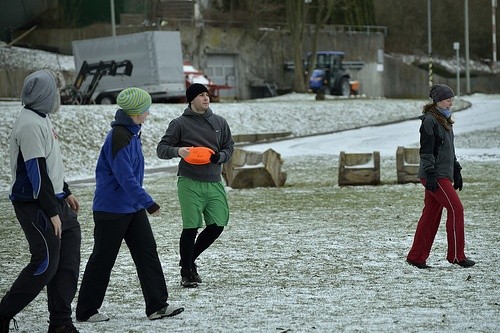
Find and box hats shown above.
[429,84,454,102]
[116,88,151,115]
[186,83,210,102]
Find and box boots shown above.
[47,268,80,333]
[0,277,41,333]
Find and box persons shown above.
[405,84,476,267]
[0,68,81,333]
[156,83,235,288]
[75,87,186,321]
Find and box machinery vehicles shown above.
[303,52,360,96]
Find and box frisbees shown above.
[184,147,215,165]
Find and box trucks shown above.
[171,65,214,103]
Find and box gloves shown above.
[425,168,439,192]
[210,150,221,163]
[452,161,463,191]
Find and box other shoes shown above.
[86,314,109,322]
[181,276,198,288]
[454,259,475,268]
[190,265,202,284]
[149,305,186,320]
[405,259,433,268]
